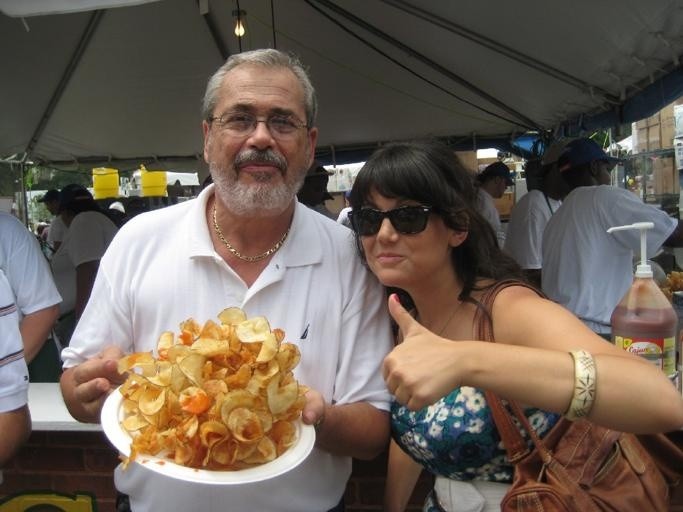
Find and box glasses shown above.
[347,204,433,236]
[209,110,311,140]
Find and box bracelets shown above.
[561,348,597,422]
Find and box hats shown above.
[486,161,516,186]
[55,184,94,218]
[541,137,570,167]
[37,189,59,202]
[569,140,620,168]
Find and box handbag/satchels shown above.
[473,279,683,510]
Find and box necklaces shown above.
[207,202,294,263]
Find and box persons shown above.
[60,46,397,512]
[540,138,683,345]
[0,208,64,366]
[473,160,516,251]
[334,189,353,230]
[352,139,683,512]
[505,140,571,280]
[33,183,127,351]
[0,268,34,486]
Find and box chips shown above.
[114,308,310,473]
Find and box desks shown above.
[27,381,105,434]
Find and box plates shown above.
[99,378,317,485]
